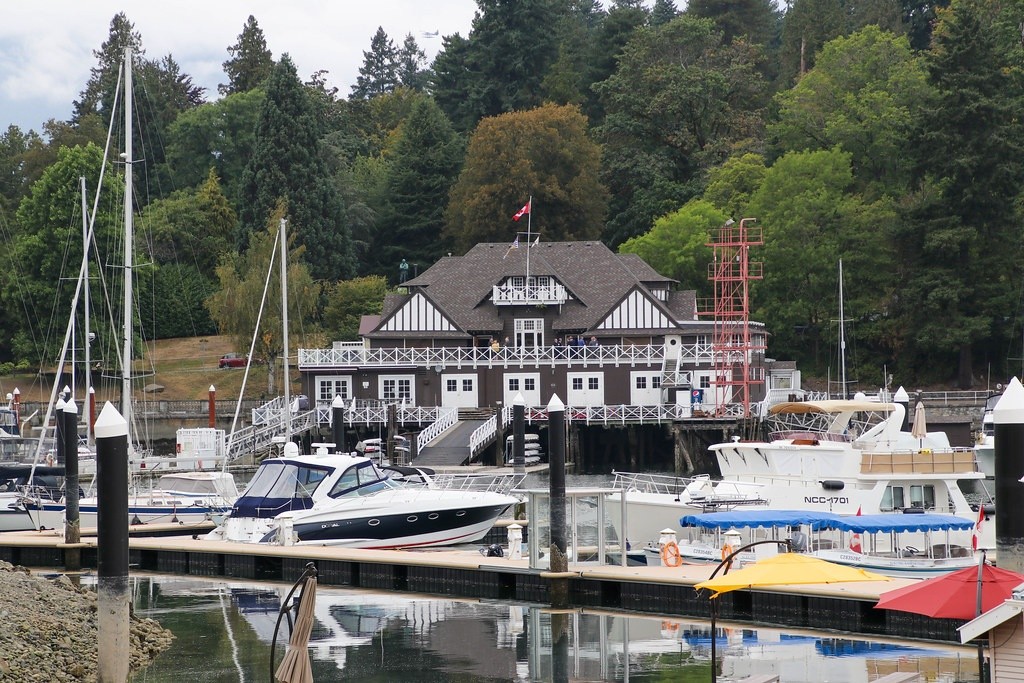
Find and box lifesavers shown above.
[664,542,680,568]
[722,544,732,570]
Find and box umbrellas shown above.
[911,401,926,448]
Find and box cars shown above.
[219,352,264,367]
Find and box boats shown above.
[207,441,527,550]
[611,254,996,568]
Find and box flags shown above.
[512,201,529,221]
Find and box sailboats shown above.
[0,46,437,538]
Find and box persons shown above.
[588,336,599,359]
[488,337,499,360]
[578,335,587,358]
[399,259,408,284]
[501,336,512,360]
[566,336,576,359]
[551,337,563,359]
